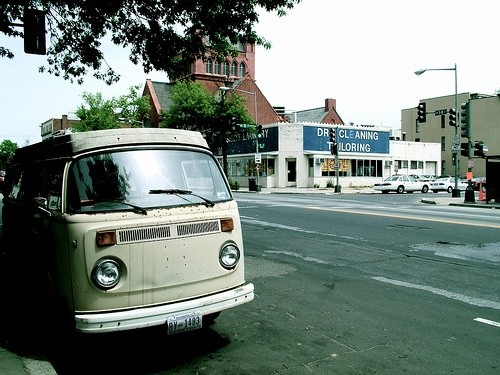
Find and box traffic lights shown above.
[256,123,262,140]
[460,98,472,140]
[448,107,457,126]
[417,102,426,123]
[229,114,238,133]
[329,128,335,142]
[461,142,471,157]
[331,143,338,155]
[474,142,484,156]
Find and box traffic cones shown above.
[477,180,485,201]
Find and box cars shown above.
[412,173,486,193]
[374,174,430,194]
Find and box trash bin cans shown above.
[465,186,475,203]
[248,177,257,191]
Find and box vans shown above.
[1,127,256,334]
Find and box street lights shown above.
[219,86,262,191]
[414,63,461,196]
